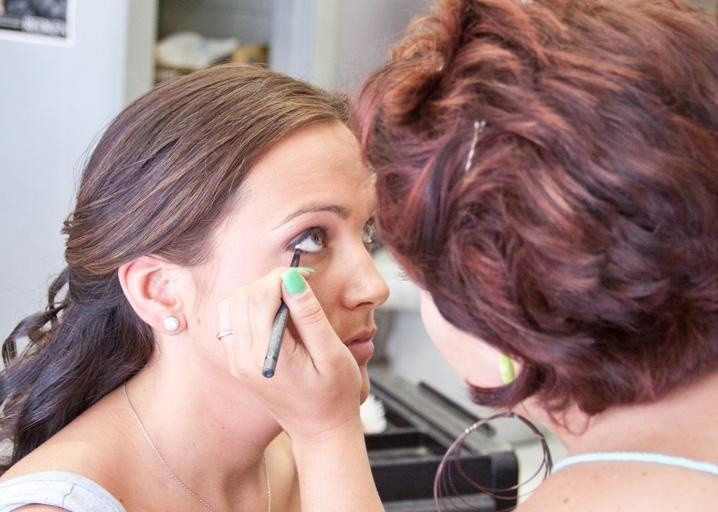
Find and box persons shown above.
[0,58,398,512]
[208,1,718,511]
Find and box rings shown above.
[214,328,233,342]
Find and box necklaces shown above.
[122,378,275,512]
